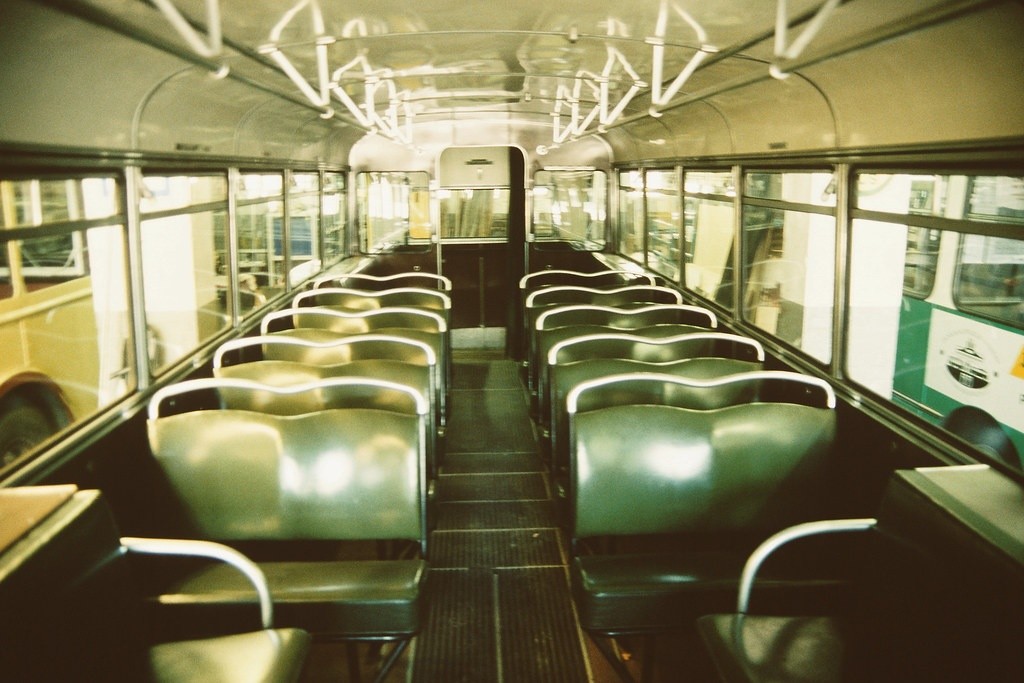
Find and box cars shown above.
[671,197,698,224]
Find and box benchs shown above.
[0,271,452,682]
[518,267,1024,682]
